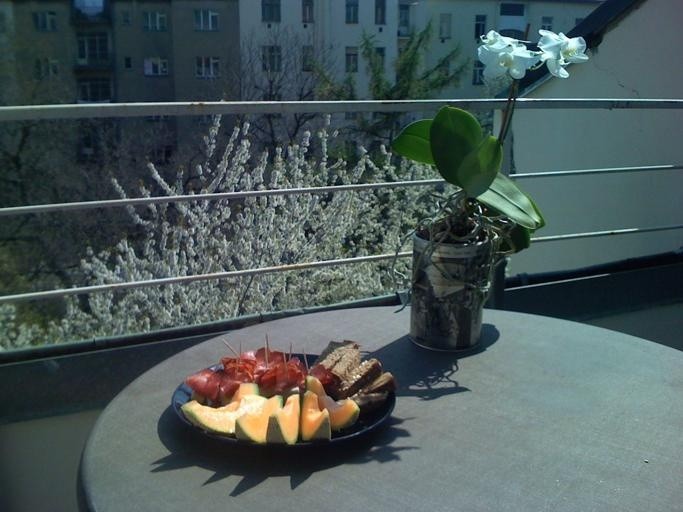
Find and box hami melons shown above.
[180,374,361,446]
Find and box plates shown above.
[171,352,398,450]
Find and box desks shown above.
[78,305,682,509]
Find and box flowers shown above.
[380,25,589,234]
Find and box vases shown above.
[412,221,498,352]
